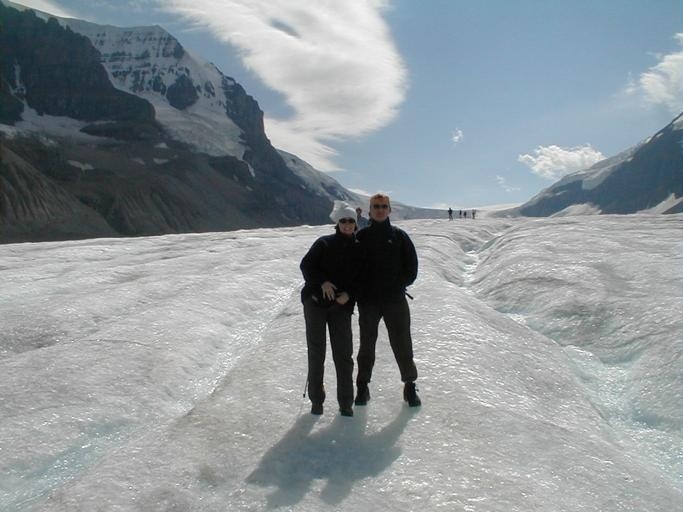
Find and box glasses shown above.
[373,204,388,208]
[339,219,355,223]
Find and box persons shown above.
[351,194,422,407]
[354,208,370,242]
[448,208,475,221]
[299,201,358,416]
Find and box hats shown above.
[336,208,357,224]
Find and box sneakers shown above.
[312,403,323,413]
[355,400,366,405]
[340,405,353,416]
[404,383,420,406]
